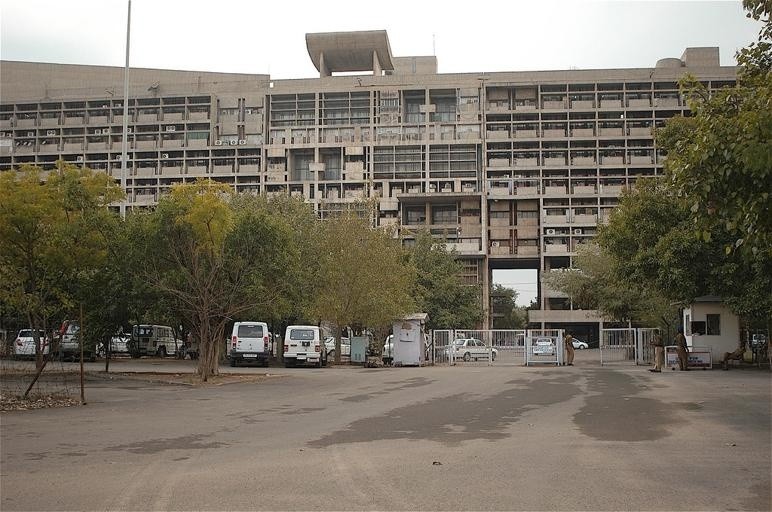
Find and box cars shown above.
[560,337,589,350]
[751,334,767,348]
[533,337,556,354]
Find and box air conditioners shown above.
[238,139,246,146]
[464,183,472,188]
[27,131,34,137]
[574,229,584,235]
[2,132,11,138]
[170,181,177,186]
[504,174,509,178]
[102,127,111,135]
[245,109,252,115]
[115,154,122,161]
[94,128,101,135]
[162,153,169,159]
[114,103,121,108]
[240,187,260,195]
[128,128,131,134]
[514,174,522,179]
[46,130,56,136]
[545,228,557,235]
[444,183,450,189]
[491,241,501,248]
[229,138,237,146]
[214,140,222,146]
[76,156,84,162]
[221,110,228,116]
[165,126,176,132]
[430,184,436,189]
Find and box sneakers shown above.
[650,369,660,372]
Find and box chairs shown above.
[731,350,746,368]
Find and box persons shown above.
[648,329,664,372]
[564,330,575,367]
[672,326,691,372]
